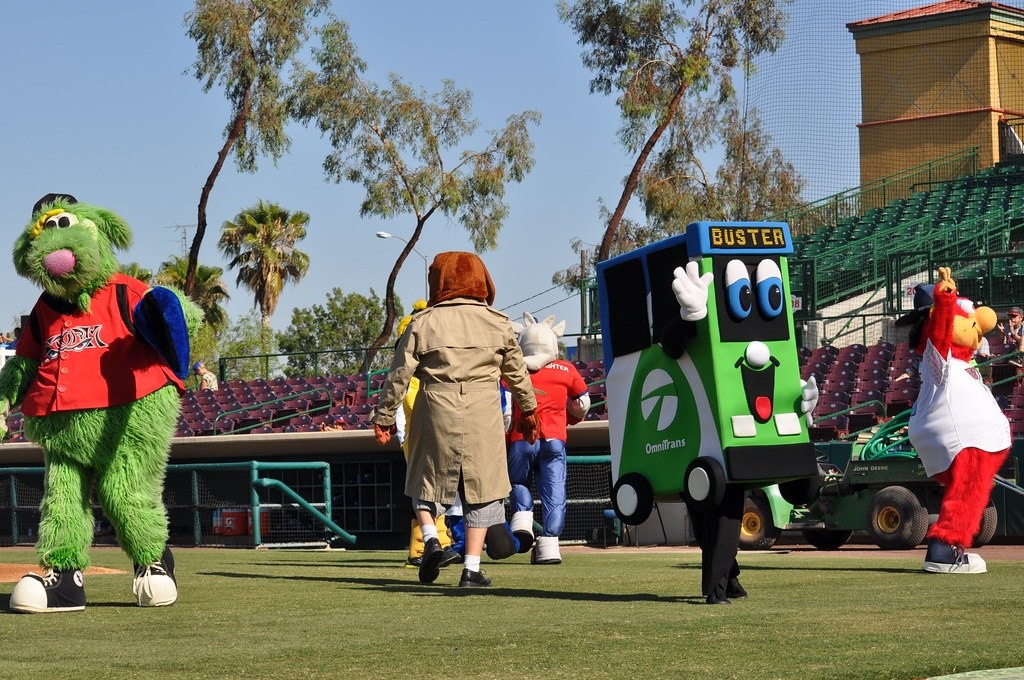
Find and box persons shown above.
[373,252,538,588]
[997,307,1024,351]
[0,327,22,350]
[974,337,990,364]
[193,362,218,392]
[596,221,819,605]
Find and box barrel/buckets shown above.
[221,508,246,536]
[248,508,269,536]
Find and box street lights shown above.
[377,231,429,301]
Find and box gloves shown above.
[374,421,397,447]
[516,411,541,445]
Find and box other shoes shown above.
[404,546,461,568]
[726,578,747,598]
[706,591,731,604]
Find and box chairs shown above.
[0,158,1024,443]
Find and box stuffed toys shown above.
[0,193,205,612]
[908,267,1014,574]
[394,300,590,565]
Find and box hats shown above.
[1006,307,1023,318]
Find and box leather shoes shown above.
[419,537,444,583]
[459,568,492,587]
